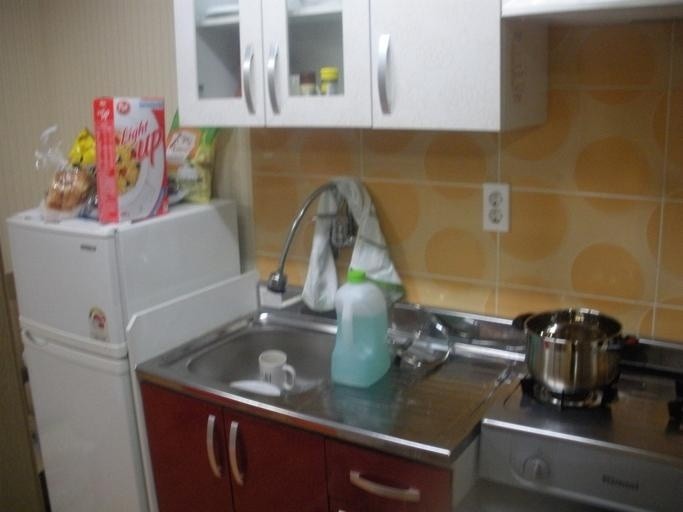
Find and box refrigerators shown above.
[5,197,240,511]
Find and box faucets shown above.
[266,182,359,293]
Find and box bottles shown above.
[299,73,316,94]
[318,67,340,93]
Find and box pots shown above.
[510,308,640,397]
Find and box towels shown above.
[302,173,405,313]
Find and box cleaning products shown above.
[330,269,392,391]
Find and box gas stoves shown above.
[483,358,682,457]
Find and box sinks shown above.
[186,324,338,398]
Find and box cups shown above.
[259,349,295,395]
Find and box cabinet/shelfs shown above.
[372,0,549,131]
[325,435,480,512]
[173,0,372,130]
[139,382,329,512]
